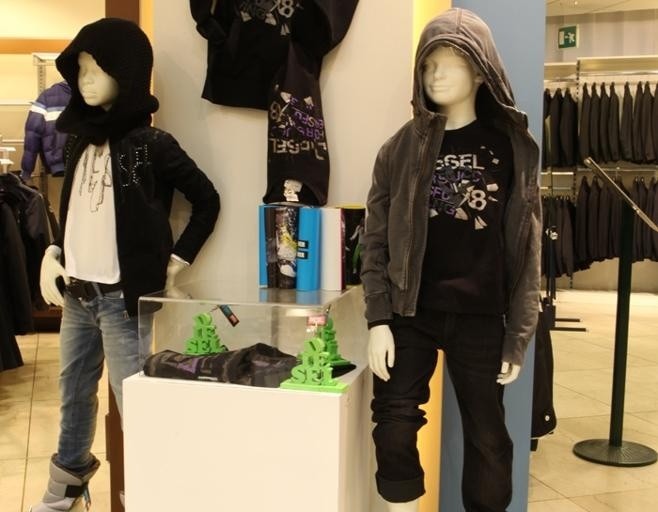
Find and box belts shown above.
[64,277,121,302]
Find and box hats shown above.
[56,18,158,145]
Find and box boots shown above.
[30,452,99,512]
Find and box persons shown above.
[359,7,544,512]
[29,16,222,512]
